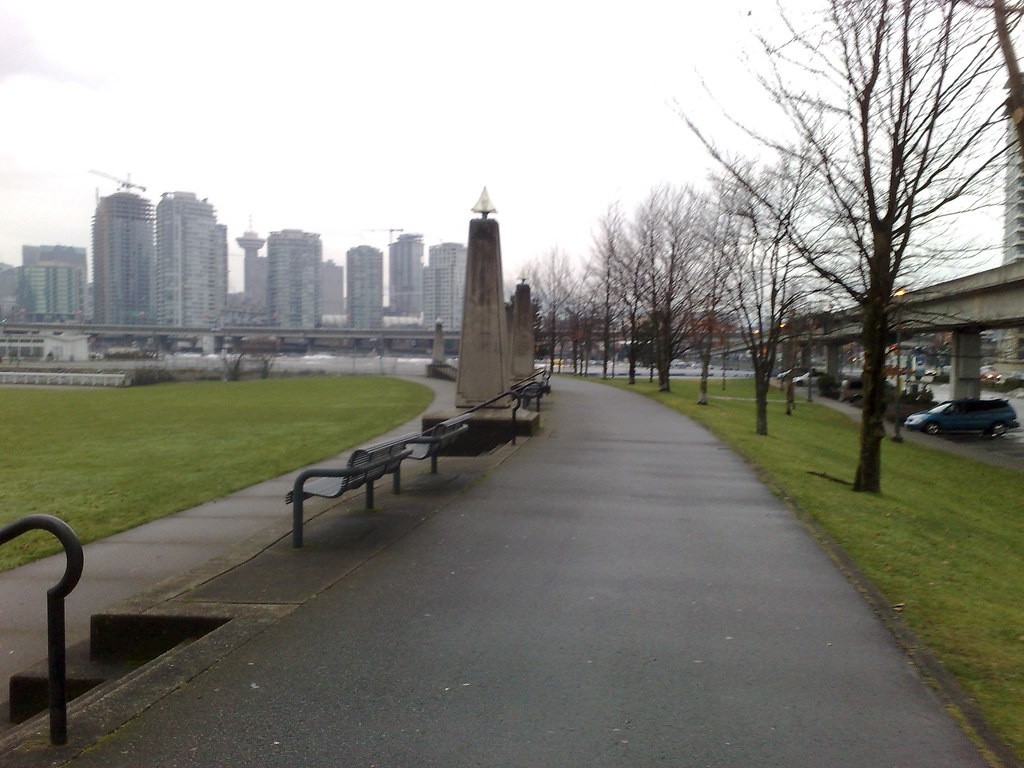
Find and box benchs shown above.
[391,411,473,494]
[523,371,551,412]
[285,433,422,549]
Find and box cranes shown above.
[368,228,403,243]
[89,169,145,194]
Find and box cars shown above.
[976,365,1023,387]
[904,398,1020,435]
[777,365,896,392]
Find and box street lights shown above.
[890,289,907,441]
[806,311,813,401]
